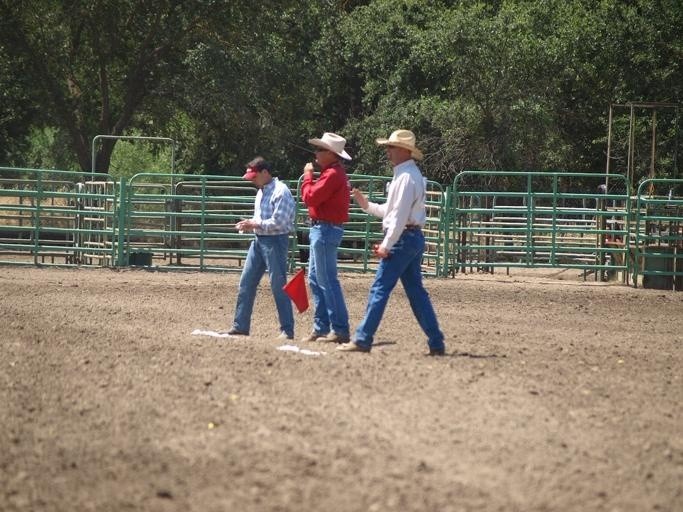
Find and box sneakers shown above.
[336,343,370,352]
[303,335,327,342]
[218,329,249,335]
[316,334,349,342]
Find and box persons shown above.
[225,154,297,340]
[336,128,445,353]
[300,131,351,343]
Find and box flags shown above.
[282,269,311,313]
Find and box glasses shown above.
[317,149,328,152]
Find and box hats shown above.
[242,158,271,180]
[309,132,352,161]
[376,130,423,160]
